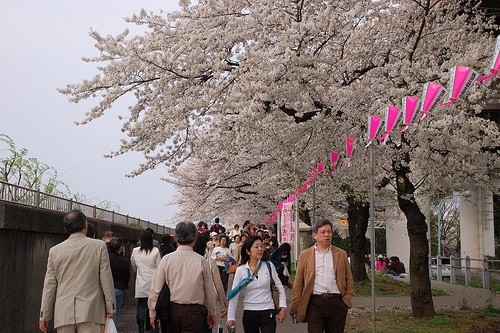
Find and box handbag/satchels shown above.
[103,316,117,333]
[266,261,282,314]
[226,258,240,273]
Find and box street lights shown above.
[437,191,473,281]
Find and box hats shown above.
[209,231,218,239]
[377,255,383,258]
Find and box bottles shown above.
[218,317,224,333]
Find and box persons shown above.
[224,234,288,333]
[129,228,162,333]
[84,216,293,297]
[39,210,116,333]
[192,232,228,333]
[147,221,217,333]
[105,237,133,330]
[365,253,407,280]
[288,218,355,333]
